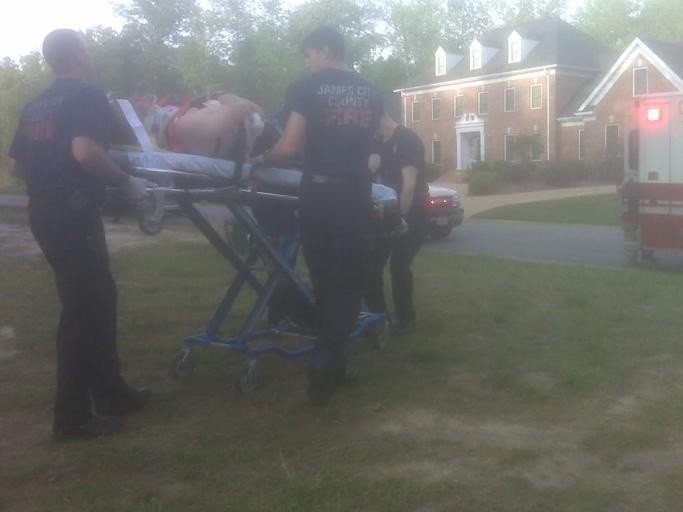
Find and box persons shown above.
[255,27,383,387]
[8,29,158,440]
[366,109,432,335]
[108,90,284,163]
[258,108,298,325]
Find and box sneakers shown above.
[53,389,152,441]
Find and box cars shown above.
[423,180,466,239]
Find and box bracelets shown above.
[263,151,271,162]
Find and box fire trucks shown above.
[620,89,682,252]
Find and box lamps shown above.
[643,107,661,123]
